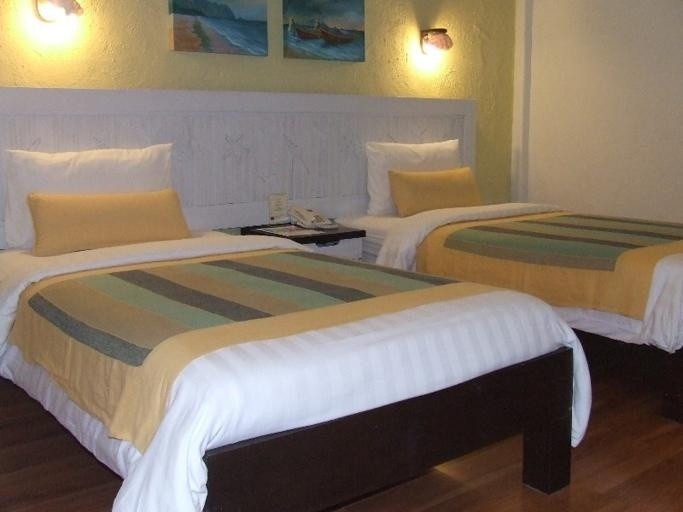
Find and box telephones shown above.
[287,205,332,228]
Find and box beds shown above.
[338,201,683,423]
[1,232,573,512]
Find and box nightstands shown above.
[241,220,365,263]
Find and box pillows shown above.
[366,139,461,217]
[3,144,173,251]
[26,190,193,256]
[387,168,483,218]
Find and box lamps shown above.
[420,28,453,54]
[36,0,84,21]
